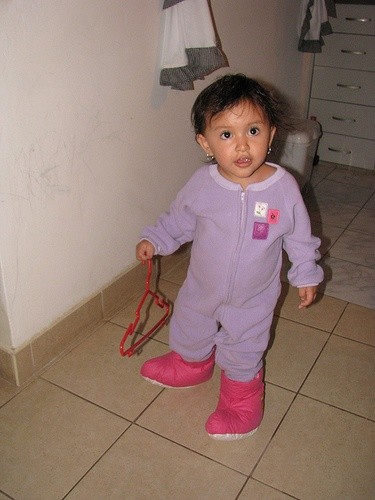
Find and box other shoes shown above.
[205,367,266,441]
[141,345,217,389]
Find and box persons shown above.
[136,73,324,441]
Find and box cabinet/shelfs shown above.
[309,3,375,171]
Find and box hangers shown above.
[120,257,169,356]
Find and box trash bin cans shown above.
[264,118,322,196]
